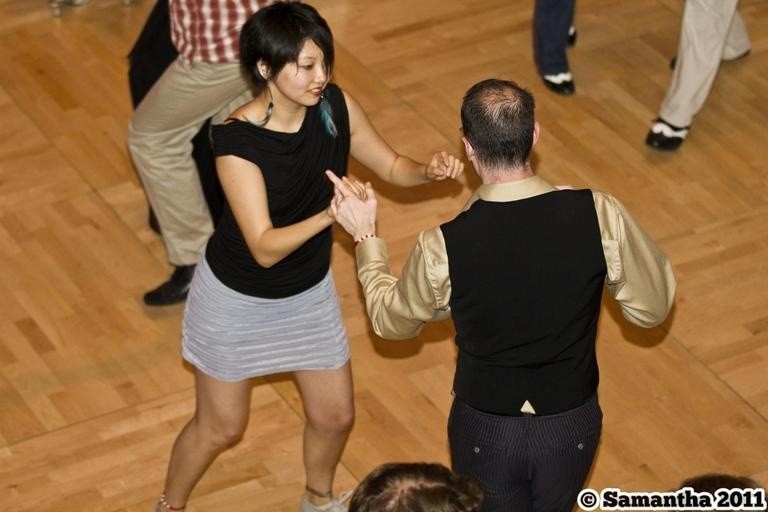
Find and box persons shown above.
[643,1,751,153]
[326,79,677,512]
[125,0,280,309]
[125,0,227,237]
[150,0,465,512]
[348,463,486,512]
[528,1,579,97]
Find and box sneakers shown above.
[299,488,355,512]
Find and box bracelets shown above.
[352,235,378,247]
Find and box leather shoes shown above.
[143,269,191,305]
[645,118,690,151]
[543,24,576,95]
